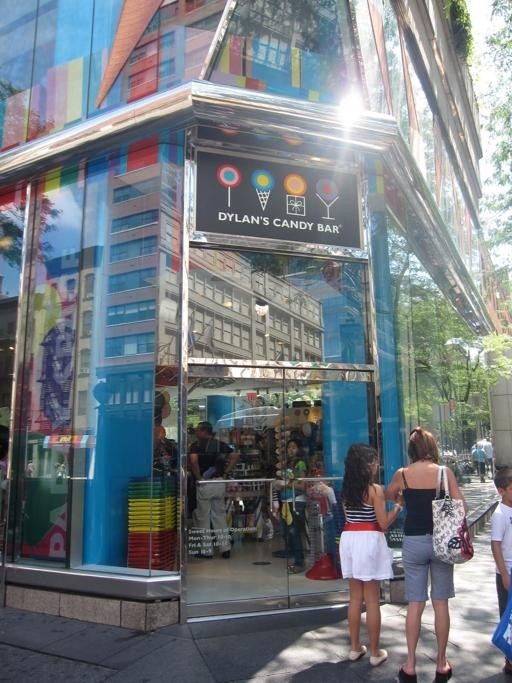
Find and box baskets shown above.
[127,476,177,571]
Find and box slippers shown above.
[399,663,417,682]
[435,662,452,682]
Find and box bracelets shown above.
[224,470,229,475]
[394,502,404,512]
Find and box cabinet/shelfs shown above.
[214,424,275,536]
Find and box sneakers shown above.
[223,551,230,558]
[350,646,367,661]
[369,649,388,666]
[288,566,305,574]
[195,554,213,559]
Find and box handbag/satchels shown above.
[432,491,474,564]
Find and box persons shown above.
[384,425,472,682]
[338,442,404,668]
[489,467,512,675]
[470,434,494,484]
[278,438,309,574]
[189,421,241,559]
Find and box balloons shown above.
[154,389,172,442]
